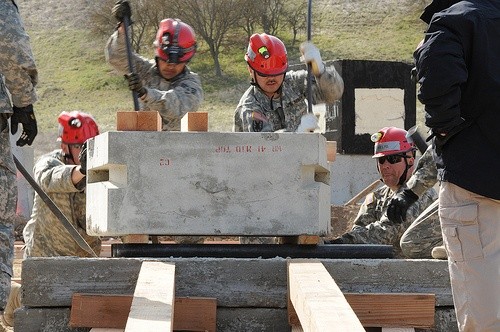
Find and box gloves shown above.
[11,104,38,146]
[387,188,418,223]
[78,142,87,175]
[295,112,321,133]
[112,1,134,30]
[299,41,324,77]
[124,73,146,98]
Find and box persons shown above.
[104,0,206,243]
[411,0,500,332]
[387,138,440,258]
[233,29,344,244]
[324,127,418,258]
[0,0,40,312]
[1,110,123,330]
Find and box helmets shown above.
[371,127,417,158]
[152,18,197,64]
[58,111,100,144]
[244,33,288,76]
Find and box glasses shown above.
[378,154,412,165]
